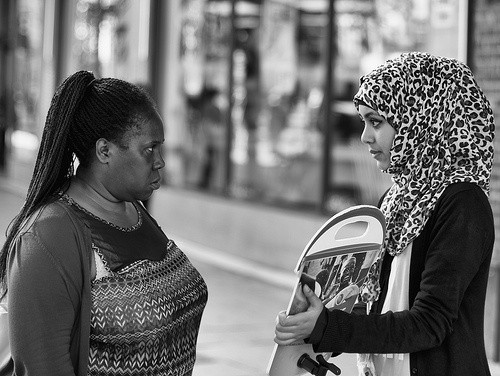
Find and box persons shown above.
[0,70,208,376]
[273,52,496,376]
[316,257,383,315]
